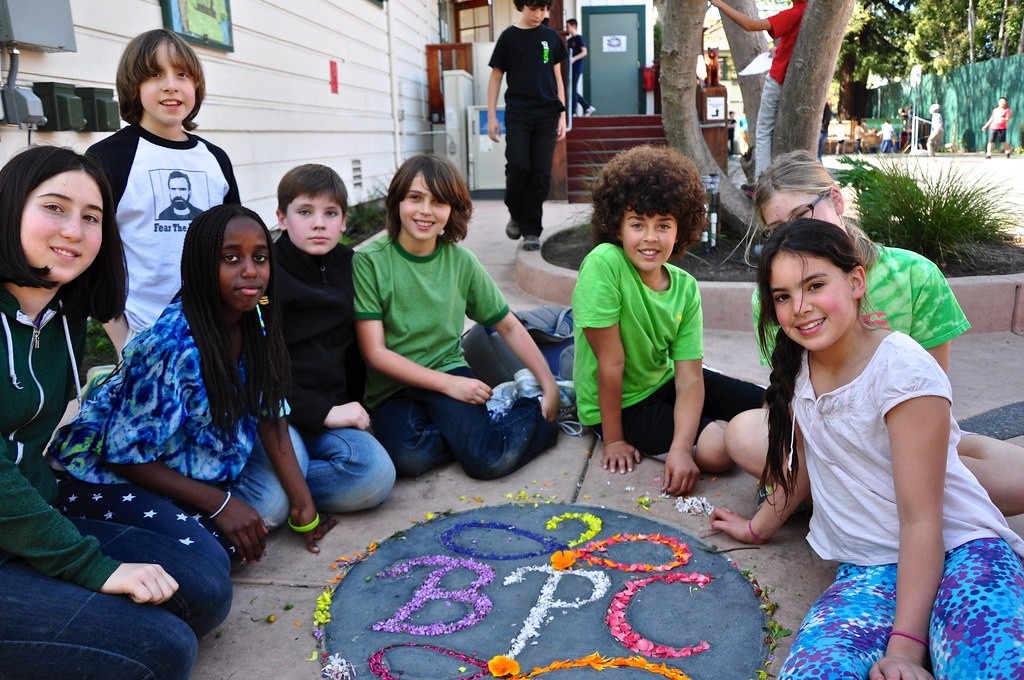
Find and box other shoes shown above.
[984,155,991,159]
[741,184,756,199]
[1006,153,1010,158]
[571,114,579,118]
[522,235,541,251]
[584,106,596,118]
[506,218,522,240]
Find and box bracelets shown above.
[210,492,231,518]
[889,631,928,645]
[288,513,320,533]
[749,521,756,541]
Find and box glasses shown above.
[761,191,831,241]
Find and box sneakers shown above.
[485,381,517,422]
[513,367,582,436]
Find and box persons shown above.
[0,144,232,680]
[881,107,924,153]
[727,111,737,153]
[566,19,596,117]
[709,218,1024,680]
[836,124,845,154]
[263,164,396,513]
[572,145,770,498]
[85,28,243,353]
[982,98,1010,159]
[740,112,749,149]
[48,204,338,567]
[724,150,1024,515]
[854,122,863,154]
[926,104,944,157]
[709,0,809,196]
[351,156,576,481]
[488,0,567,250]
[818,97,832,160]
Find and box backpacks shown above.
[463,305,574,389]
